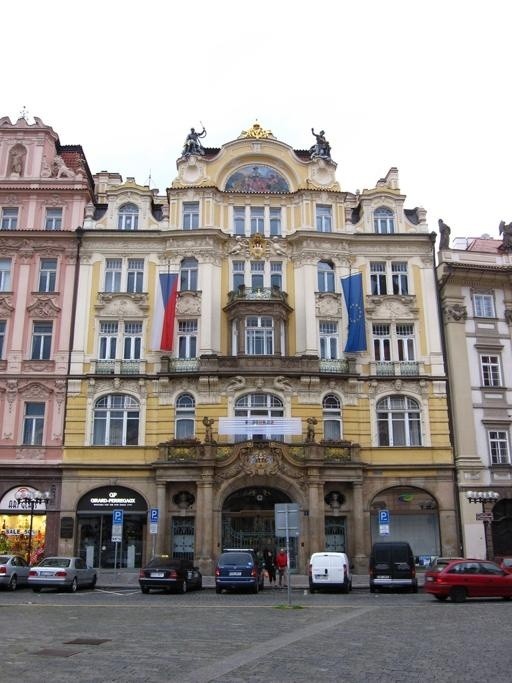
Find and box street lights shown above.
[466,490,500,560]
[14,490,51,564]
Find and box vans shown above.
[369,541,417,593]
[309,551,352,594]
[215,548,264,594]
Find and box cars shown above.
[425,555,512,603]
[0,555,97,593]
[139,560,202,594]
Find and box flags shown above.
[340,273,368,352]
[158,272,179,352]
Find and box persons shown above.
[276,547,288,587]
[184,128,206,155]
[310,127,328,156]
[264,549,278,584]
[241,166,283,192]
[18,534,27,561]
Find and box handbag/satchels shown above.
[262,569,269,578]
